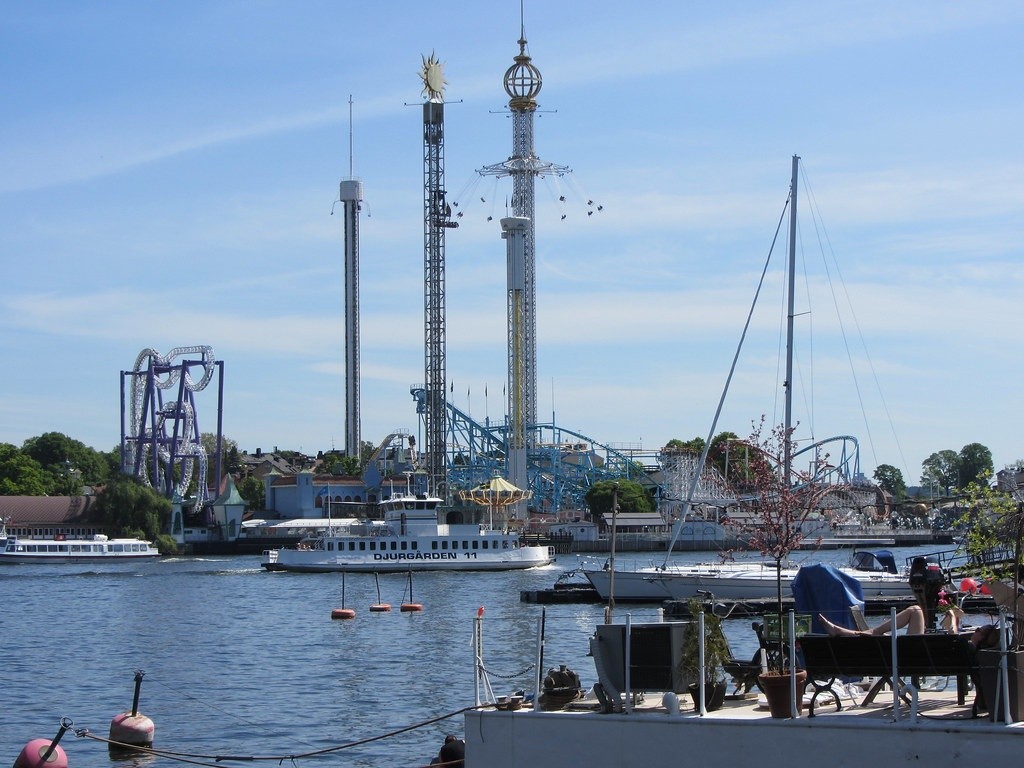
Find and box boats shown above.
[518,586,596,604]
[261,472,557,573]
[0,510,163,564]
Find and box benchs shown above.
[799,633,989,718]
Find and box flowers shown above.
[937,590,954,612]
[679,413,891,672]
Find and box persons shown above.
[908,555,963,630]
[838,509,934,532]
[818,605,1000,651]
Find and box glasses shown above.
[976,627,984,635]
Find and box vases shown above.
[759,667,805,719]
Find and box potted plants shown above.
[676,598,730,713]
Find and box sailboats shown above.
[582,153,983,602]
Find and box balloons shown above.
[959,578,977,592]
[981,578,994,595]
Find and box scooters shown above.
[691,589,802,696]
